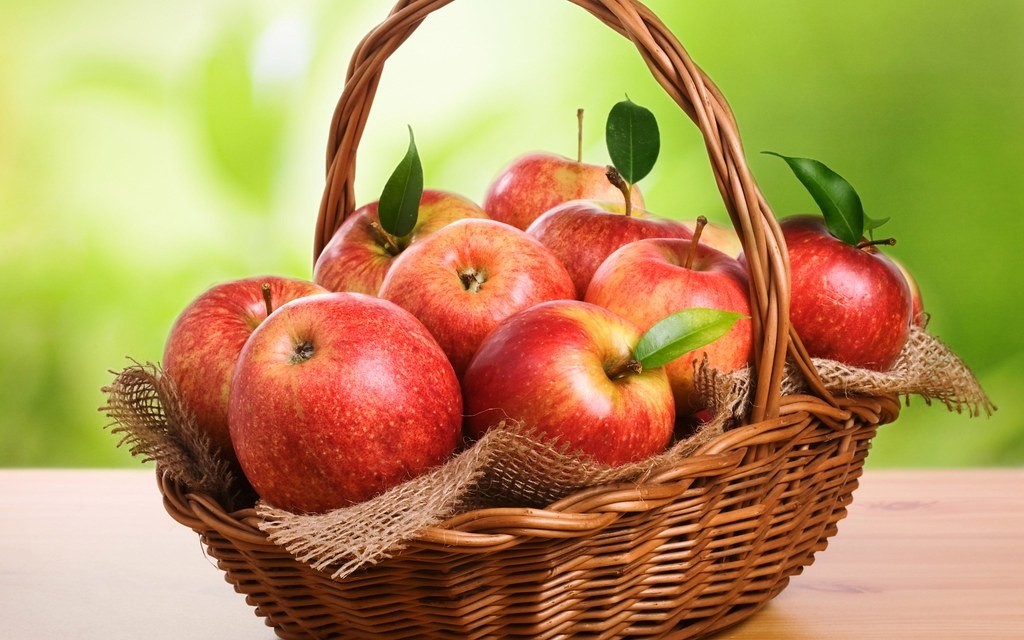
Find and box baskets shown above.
[155,0,901,640]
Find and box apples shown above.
[159,91,926,513]
